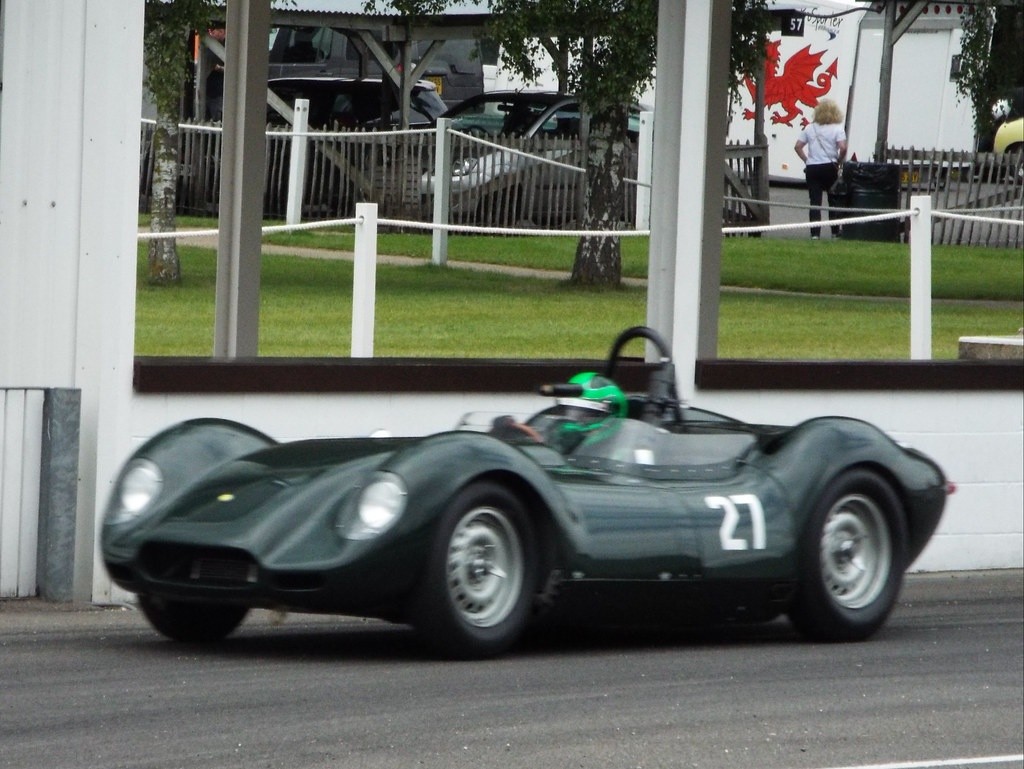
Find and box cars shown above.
[425,90,639,217]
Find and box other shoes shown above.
[812,236,819,240]
[830,230,842,240]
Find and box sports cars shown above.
[101,326,956,658]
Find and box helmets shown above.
[557,371,628,453]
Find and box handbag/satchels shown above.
[831,177,845,195]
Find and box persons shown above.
[192,20,226,128]
[794,99,848,240]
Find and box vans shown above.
[261,76,448,216]
